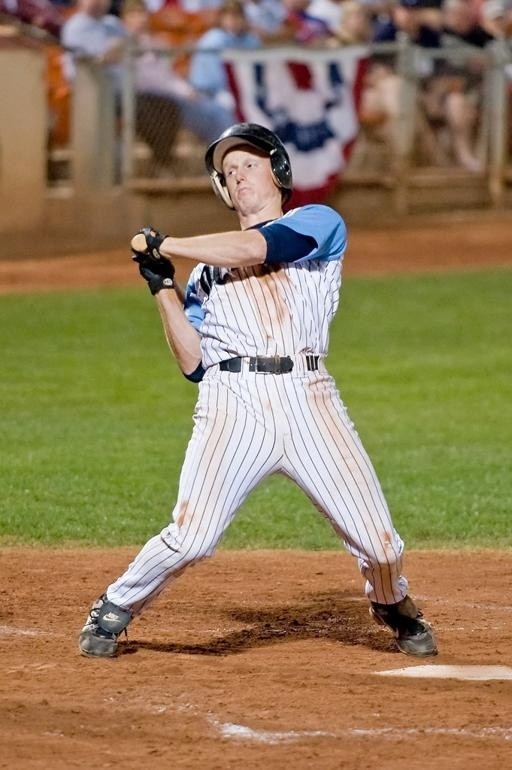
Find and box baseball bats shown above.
[131,232,187,303]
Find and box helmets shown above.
[205,123,292,210]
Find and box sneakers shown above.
[369,594,437,657]
[79,594,133,657]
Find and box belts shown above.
[219,356,319,373]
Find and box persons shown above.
[80,123,438,659]
[0,0,512,180]
